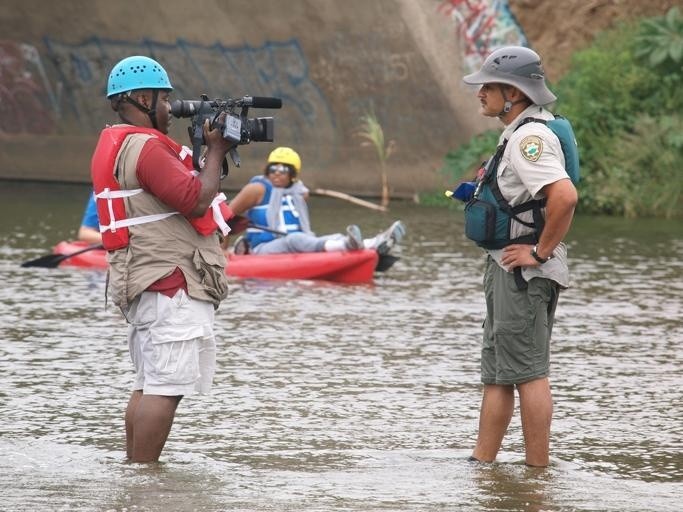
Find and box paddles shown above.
[219,215,400,271]
[21,242,103,268]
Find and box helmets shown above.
[461,46,558,107]
[106,55,175,99]
[267,146,302,175]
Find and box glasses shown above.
[267,165,291,174]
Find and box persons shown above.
[217,146,405,255]
[89,53,241,469]
[76,191,249,257]
[444,43,585,472]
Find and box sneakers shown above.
[343,224,366,250]
[373,219,407,257]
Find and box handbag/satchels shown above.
[464,156,513,251]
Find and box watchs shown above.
[527,243,549,264]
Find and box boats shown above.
[54,239,378,288]
